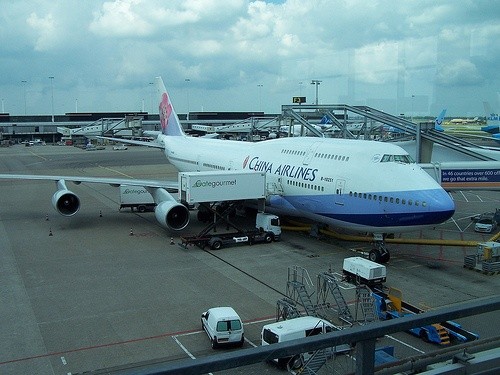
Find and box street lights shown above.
[49,76,55,144]
[412,95,415,117]
[258,84,264,111]
[297,82,304,111]
[149,82,154,114]
[185,79,190,120]
[20,80,27,115]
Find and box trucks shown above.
[179,212,283,250]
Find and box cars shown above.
[474,218,498,234]
[58,139,128,151]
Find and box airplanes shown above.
[379,108,447,138]
[56,112,372,138]
[0,74,500,266]
[449,115,479,125]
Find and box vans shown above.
[260,315,342,371]
[201,307,245,350]
